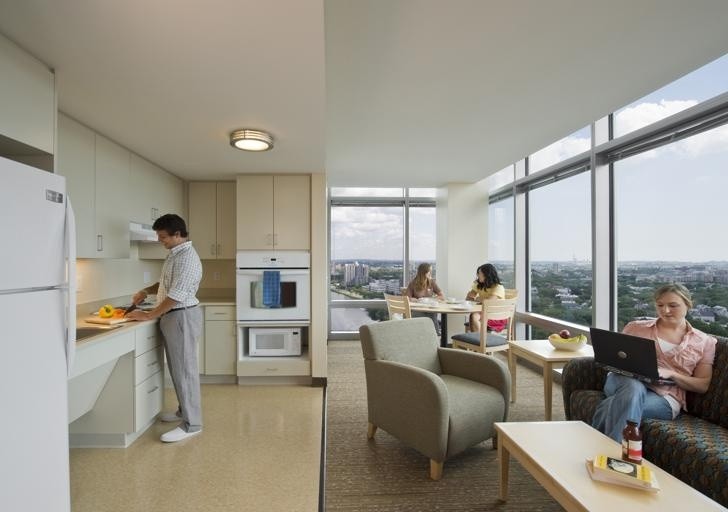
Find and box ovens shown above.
[236,251,311,322]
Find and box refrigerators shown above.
[0,156,76,511]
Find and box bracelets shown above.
[139,289,148,298]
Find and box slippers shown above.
[160,424,203,443]
[161,413,183,422]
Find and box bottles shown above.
[622,418,643,464]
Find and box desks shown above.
[505,338,594,420]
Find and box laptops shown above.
[590,327,676,386]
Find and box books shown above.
[580,451,663,497]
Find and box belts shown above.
[162,303,199,315]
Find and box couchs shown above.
[561,332,727,510]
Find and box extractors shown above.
[130,221,159,243]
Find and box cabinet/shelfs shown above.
[237,173,311,252]
[57,114,132,260]
[164,306,234,377]
[1,36,57,157]
[130,153,185,231]
[67,321,163,429]
[188,182,237,260]
[236,357,311,387]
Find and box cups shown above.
[410,297,474,311]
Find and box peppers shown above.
[98,304,112,318]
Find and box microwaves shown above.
[248,329,302,357]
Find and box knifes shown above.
[123,299,144,315]
[114,300,154,312]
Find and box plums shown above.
[559,329,570,338]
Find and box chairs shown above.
[360,316,511,480]
[384,285,519,403]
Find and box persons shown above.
[465,263,508,334]
[124,211,205,443]
[591,277,718,440]
[405,261,445,340]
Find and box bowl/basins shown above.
[547,338,586,350]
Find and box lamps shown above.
[229,130,272,152]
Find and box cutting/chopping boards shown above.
[86,316,133,325]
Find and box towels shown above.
[262,270,281,307]
